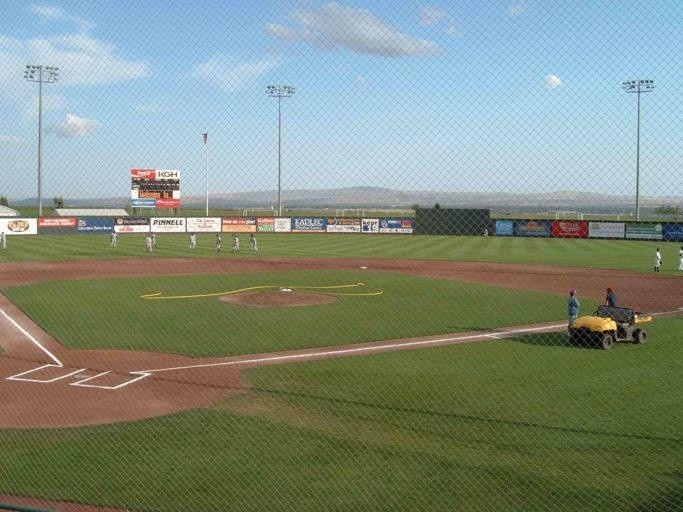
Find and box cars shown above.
[568,304,652,349]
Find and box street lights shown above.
[24,64,60,216]
[265,85,295,217]
[620,79,656,220]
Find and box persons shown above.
[676,246,683,271]
[110,230,118,249]
[188,232,198,253]
[654,247,663,273]
[567,288,580,336]
[215,234,222,254]
[232,233,240,254]
[145,234,152,254]
[604,286,617,308]
[150,232,156,248]
[247,233,260,258]
